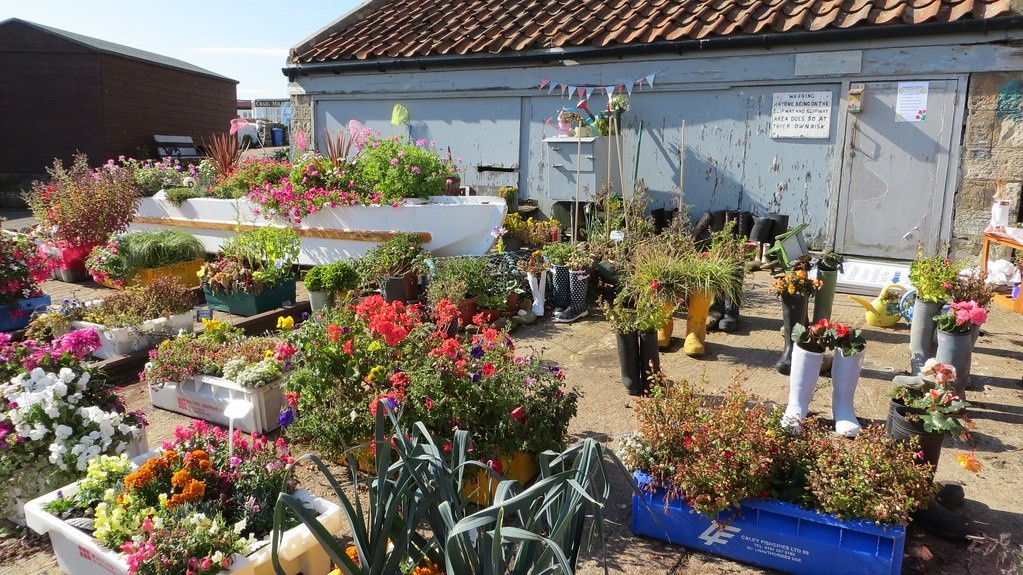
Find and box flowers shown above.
[0,97,753,574]
[619,361,933,529]
[769,269,824,297]
[896,360,985,475]
[933,300,988,334]
[829,322,867,355]
[790,319,833,351]
[910,241,966,302]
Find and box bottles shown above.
[993,199,1010,228]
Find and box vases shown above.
[774,291,809,375]
[782,342,826,435]
[831,347,864,437]
[95,256,205,292]
[884,399,942,478]
[46,297,199,363]
[1,426,149,525]
[910,294,946,372]
[0,293,50,331]
[893,321,973,403]
[23,448,341,574]
[117,187,508,278]
[200,278,297,317]
[330,422,538,507]
[4,226,107,283]
[142,361,293,435]
[631,466,908,574]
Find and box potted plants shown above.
[811,249,845,326]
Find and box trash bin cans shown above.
[271,128,283,146]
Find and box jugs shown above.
[848,284,908,328]
[900,289,951,324]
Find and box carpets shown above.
[808,259,913,296]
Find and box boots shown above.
[886,395,907,434]
[650,312,673,350]
[639,330,668,397]
[936,324,972,402]
[684,284,712,356]
[617,333,641,394]
[718,274,742,331]
[650,207,679,238]
[776,293,811,374]
[706,276,724,330]
[831,344,866,438]
[555,270,589,323]
[779,342,825,436]
[910,296,939,381]
[890,406,943,491]
[812,259,837,338]
[550,265,570,315]
[527,269,547,316]
[690,210,789,263]
